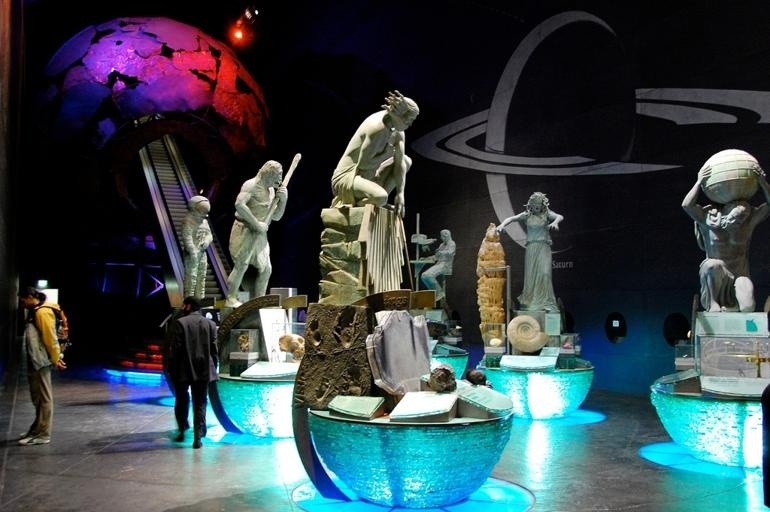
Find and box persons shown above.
[331,90,420,219]
[421,229,456,301]
[160,295,220,449]
[494,191,564,313]
[181,195,215,300]
[15,287,67,445]
[681,162,770,313]
[224,160,288,309]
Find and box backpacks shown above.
[39,301,68,352]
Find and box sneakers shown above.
[19,432,50,445]
[174,436,203,448]
